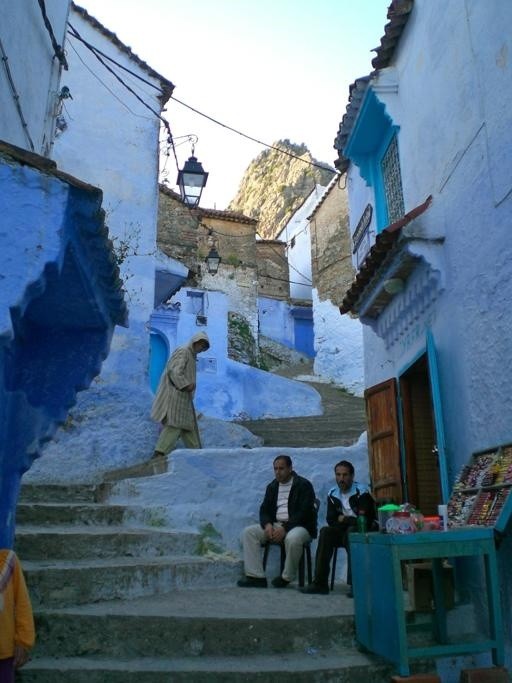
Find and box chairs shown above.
[264,499,319,585]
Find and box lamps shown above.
[177,143,208,210]
[205,247,222,274]
[385,279,404,294]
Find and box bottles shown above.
[356,511,367,537]
[377,503,448,535]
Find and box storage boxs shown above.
[406,561,455,609]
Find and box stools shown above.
[329,542,352,593]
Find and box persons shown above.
[149,328,212,459]
[298,459,378,599]
[0,545,37,683]
[235,454,317,587]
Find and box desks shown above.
[347,529,505,677]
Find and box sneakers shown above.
[237,576,268,588]
[271,576,290,587]
[301,581,330,594]
[346,585,353,598]
